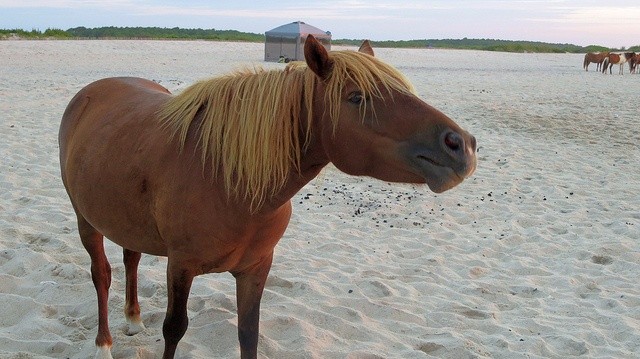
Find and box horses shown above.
[583,50,640,75]
[56,34,478,359]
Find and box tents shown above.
[265,21,331,64]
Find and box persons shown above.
[278,55,296,63]
